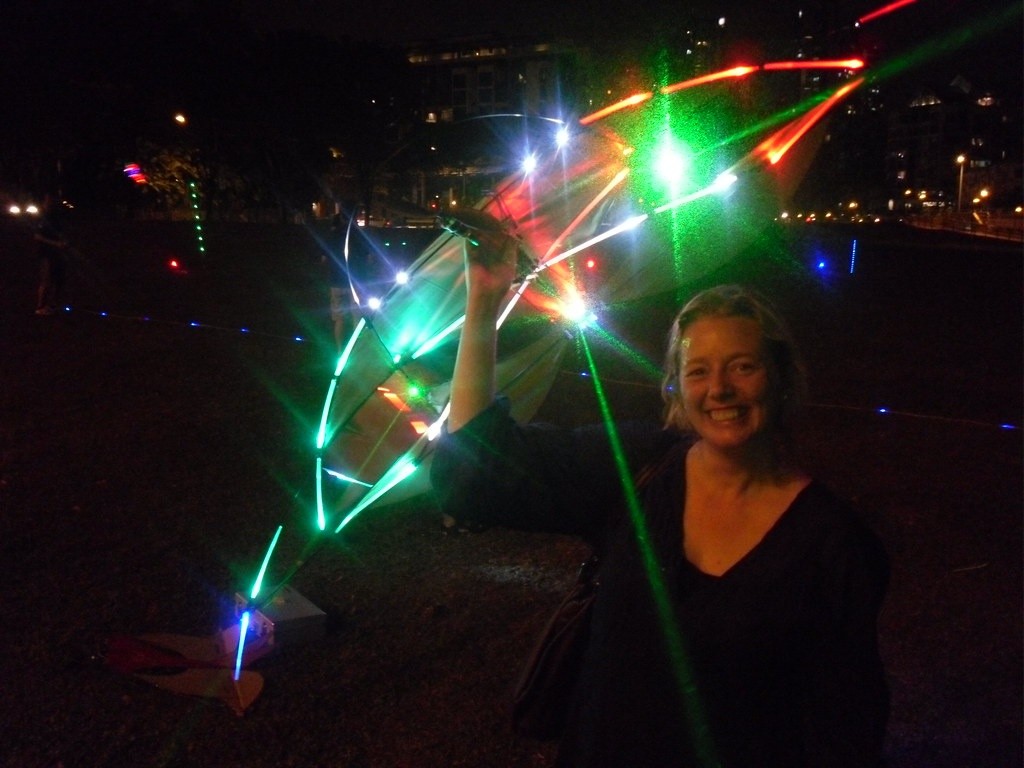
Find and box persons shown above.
[431,217,894,768]
[34,217,64,317]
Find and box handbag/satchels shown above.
[512,433,701,742]
